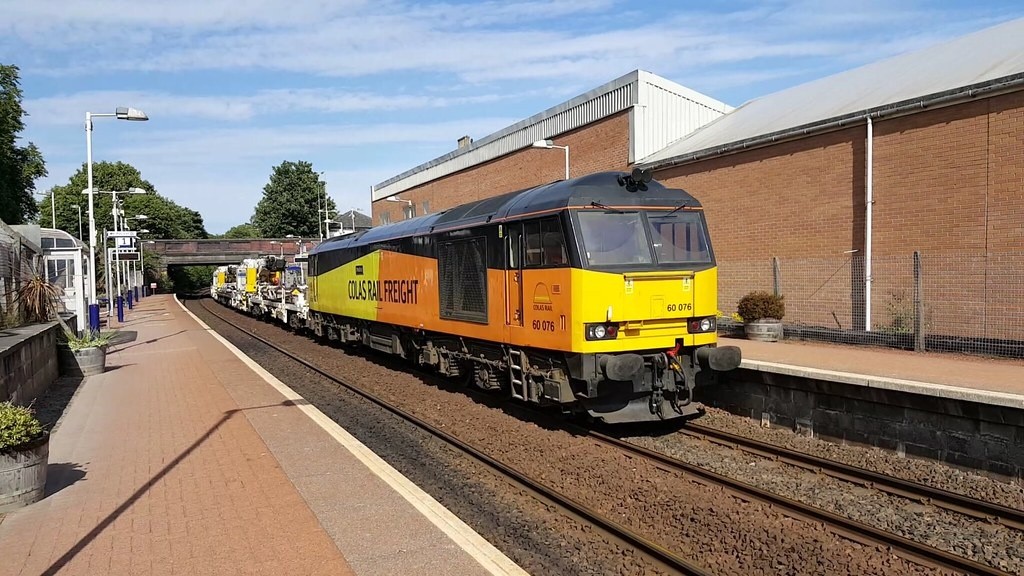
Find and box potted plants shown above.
[737,289,786,342]
[56,325,119,376]
[0,400,50,515]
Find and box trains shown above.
[209,169,742,425]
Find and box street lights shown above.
[385,196,413,220]
[323,219,343,236]
[269,241,283,259]
[531,139,569,181]
[84,106,148,341]
[37,190,59,277]
[66,186,157,329]
[286,234,304,285]
